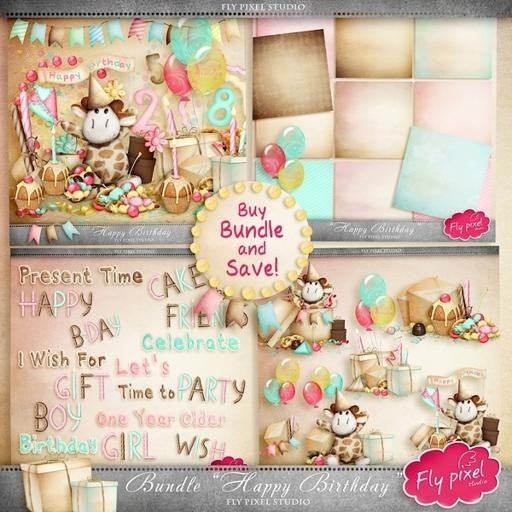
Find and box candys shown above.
[66,166,159,217]
[192,191,213,202]
[451,314,500,343]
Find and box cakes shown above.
[429,294,461,336]
[160,177,194,215]
[13,181,45,210]
[38,162,70,197]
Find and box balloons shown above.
[277,159,304,193]
[324,371,345,397]
[187,46,227,97]
[263,378,282,407]
[260,144,288,178]
[368,294,397,329]
[278,380,296,404]
[311,366,331,389]
[303,380,323,408]
[162,52,195,101]
[275,357,300,384]
[354,300,375,332]
[358,273,387,309]
[170,17,214,67]
[275,126,306,161]
